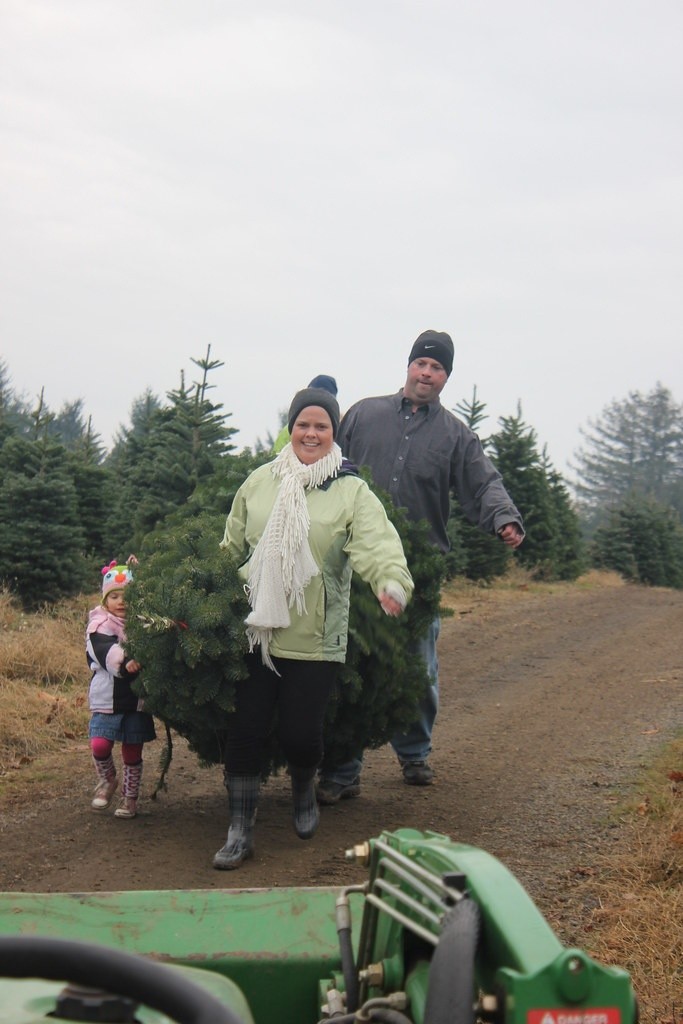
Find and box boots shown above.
[213,770,262,870]
[288,752,321,839]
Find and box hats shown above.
[101,554,138,609]
[408,330,454,379]
[288,375,340,440]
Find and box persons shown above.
[309,328,525,803]
[82,556,157,818]
[214,374,415,874]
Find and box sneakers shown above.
[91,774,119,810]
[114,797,136,818]
[316,775,361,803]
[397,757,432,785]
